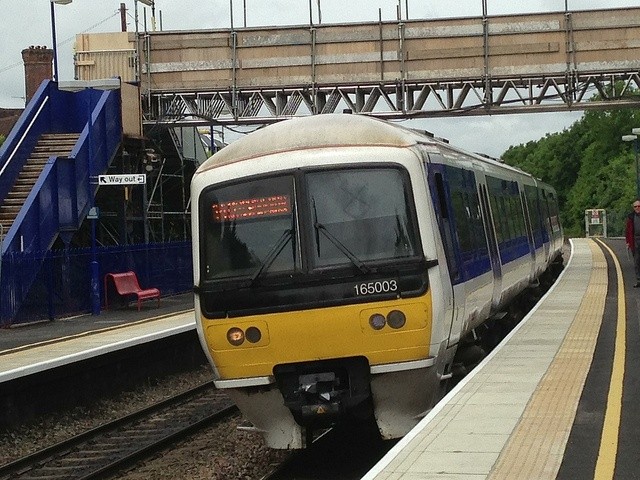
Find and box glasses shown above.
[633,204,640,209]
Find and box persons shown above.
[625,198,640,288]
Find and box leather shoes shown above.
[633,283,639,288]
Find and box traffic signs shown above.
[99,174,147,185]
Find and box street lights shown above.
[50,0,73,85]
[622,135,639,203]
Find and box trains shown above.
[189,113,564,451]
